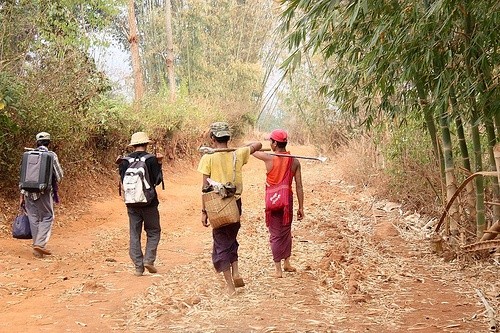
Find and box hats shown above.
[210,122,231,137]
[267,130,287,142]
[35,132,50,141]
[129,132,150,145]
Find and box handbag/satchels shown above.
[265,184,289,210]
[12,206,33,239]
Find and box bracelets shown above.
[202,209,206,212]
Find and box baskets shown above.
[201,191,240,229]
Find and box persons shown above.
[198,122,262,294]
[251,129,304,277]
[19,132,63,259]
[119,132,165,276]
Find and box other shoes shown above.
[269,272,282,278]
[33,249,44,258]
[33,245,53,255]
[234,278,245,287]
[144,263,157,272]
[132,268,143,275]
[225,281,237,298]
[284,267,296,272]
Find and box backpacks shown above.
[119,154,155,208]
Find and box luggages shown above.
[18,146,53,195]
